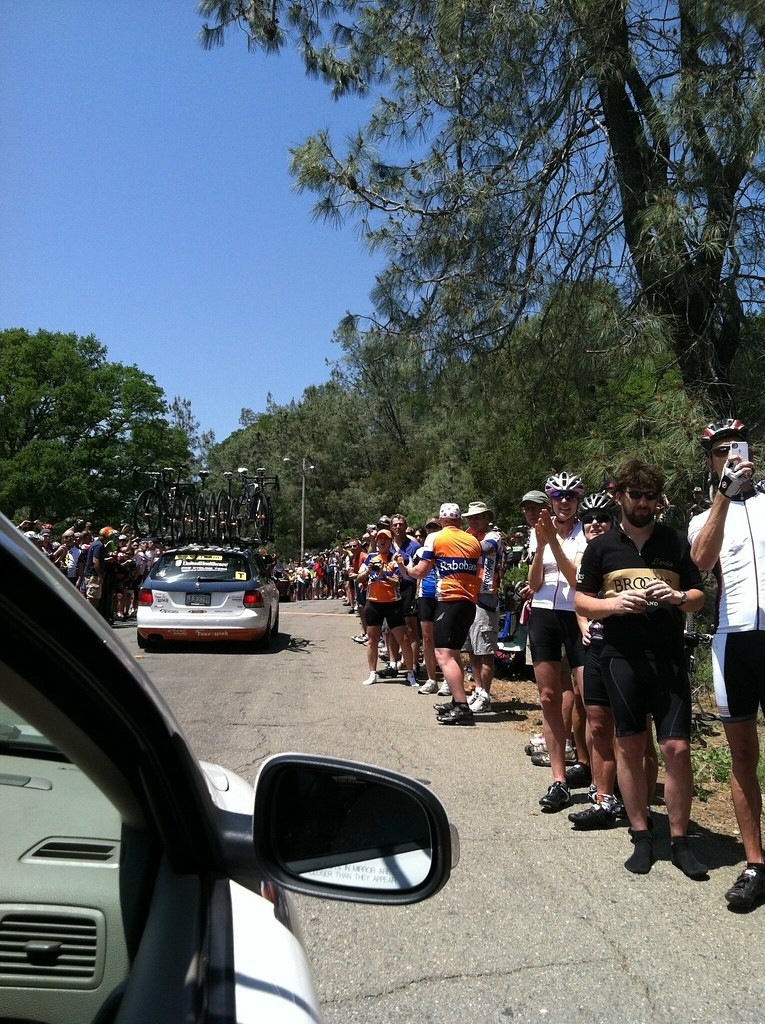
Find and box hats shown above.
[424,517,442,528]
[518,489,549,509]
[40,529,52,538]
[98,527,117,542]
[439,502,462,519]
[377,528,394,540]
[461,501,494,522]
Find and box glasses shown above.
[414,534,424,538]
[406,532,411,535]
[583,514,612,524]
[349,545,356,548]
[622,489,659,500]
[392,523,404,527]
[710,445,730,458]
[549,490,581,501]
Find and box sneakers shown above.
[524,731,764,909]
[351,632,450,697]
[433,701,475,725]
[467,691,491,713]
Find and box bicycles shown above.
[130,465,282,546]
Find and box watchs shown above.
[676,591,687,606]
[406,567,412,577]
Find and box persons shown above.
[573,460,709,879]
[15,519,171,626]
[688,418,765,909]
[283,473,657,838]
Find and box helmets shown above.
[25,530,39,540]
[544,471,584,498]
[698,418,749,459]
[578,493,621,522]
[118,534,129,543]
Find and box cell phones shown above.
[730,442,749,466]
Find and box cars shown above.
[135,544,289,646]
[0,515,461,1024]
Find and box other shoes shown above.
[341,599,360,617]
[113,611,136,622]
[314,594,346,600]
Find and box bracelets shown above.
[19,525,22,530]
